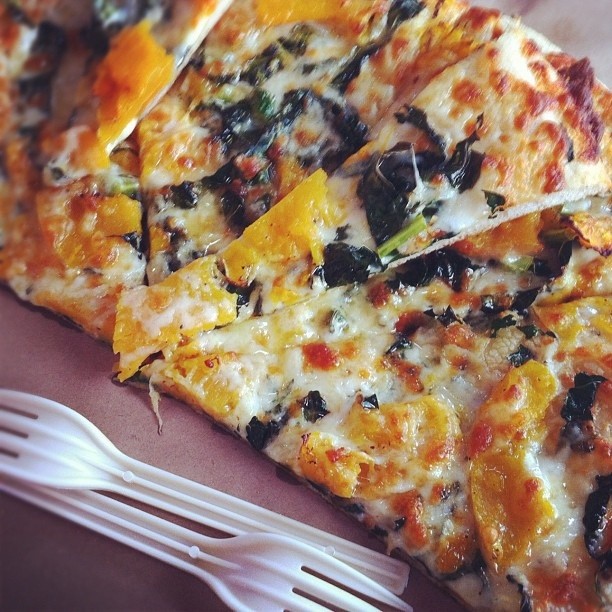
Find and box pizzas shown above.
[1,0,611,612]
